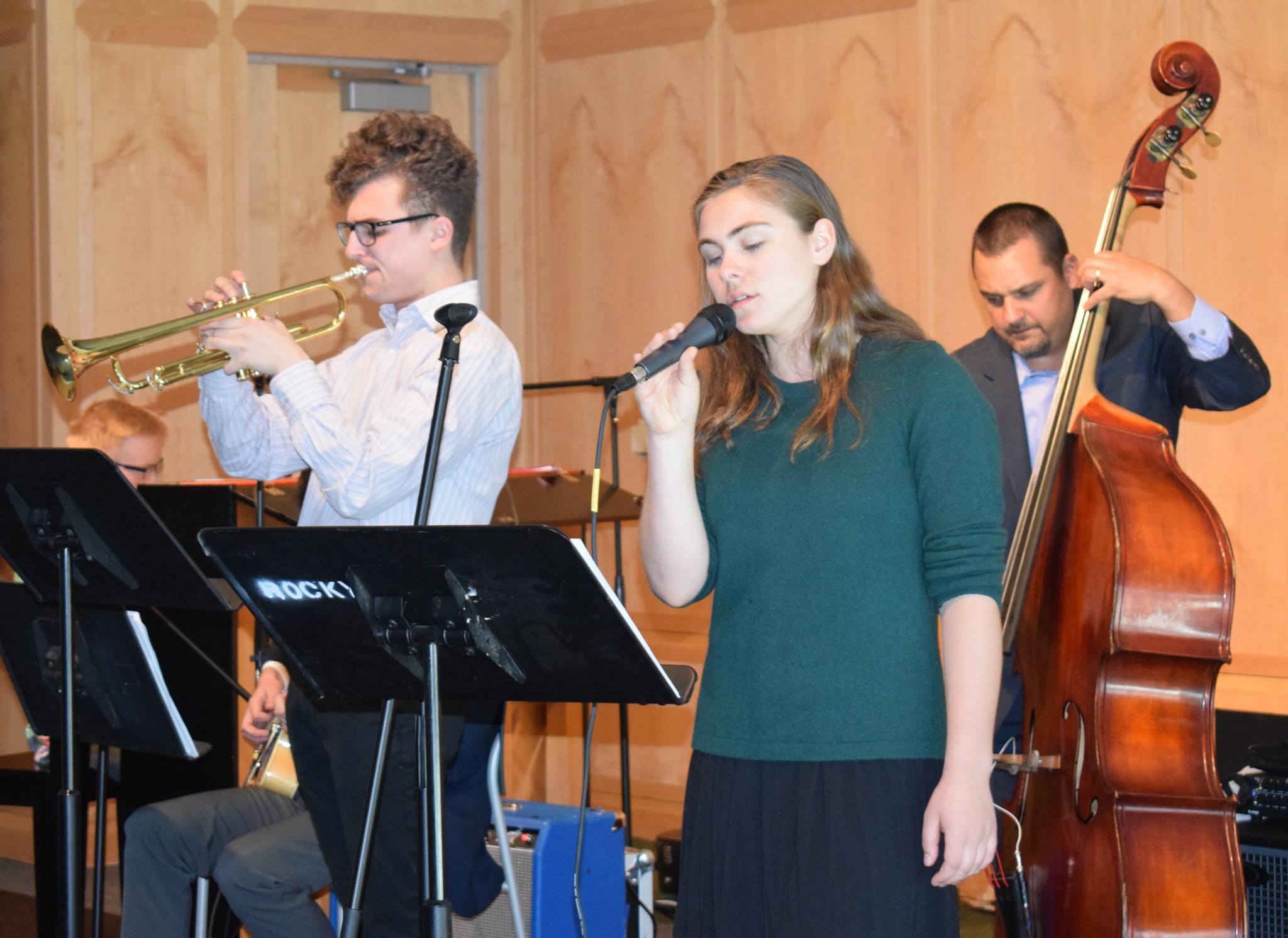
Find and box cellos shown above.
[982,40,1252,936]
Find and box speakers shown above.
[1231,813,1288,938]
[329,799,630,938]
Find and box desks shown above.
[0,748,157,938]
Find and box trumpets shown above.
[41,262,369,404]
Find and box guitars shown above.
[238,716,300,800]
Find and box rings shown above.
[1096,268,1100,279]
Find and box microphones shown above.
[610,304,736,395]
[1242,862,1268,885]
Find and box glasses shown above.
[334,213,441,247]
[114,458,164,479]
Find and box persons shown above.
[120,642,338,938]
[67,399,169,488]
[635,155,1007,938]
[952,203,1271,806]
[187,110,523,938]
